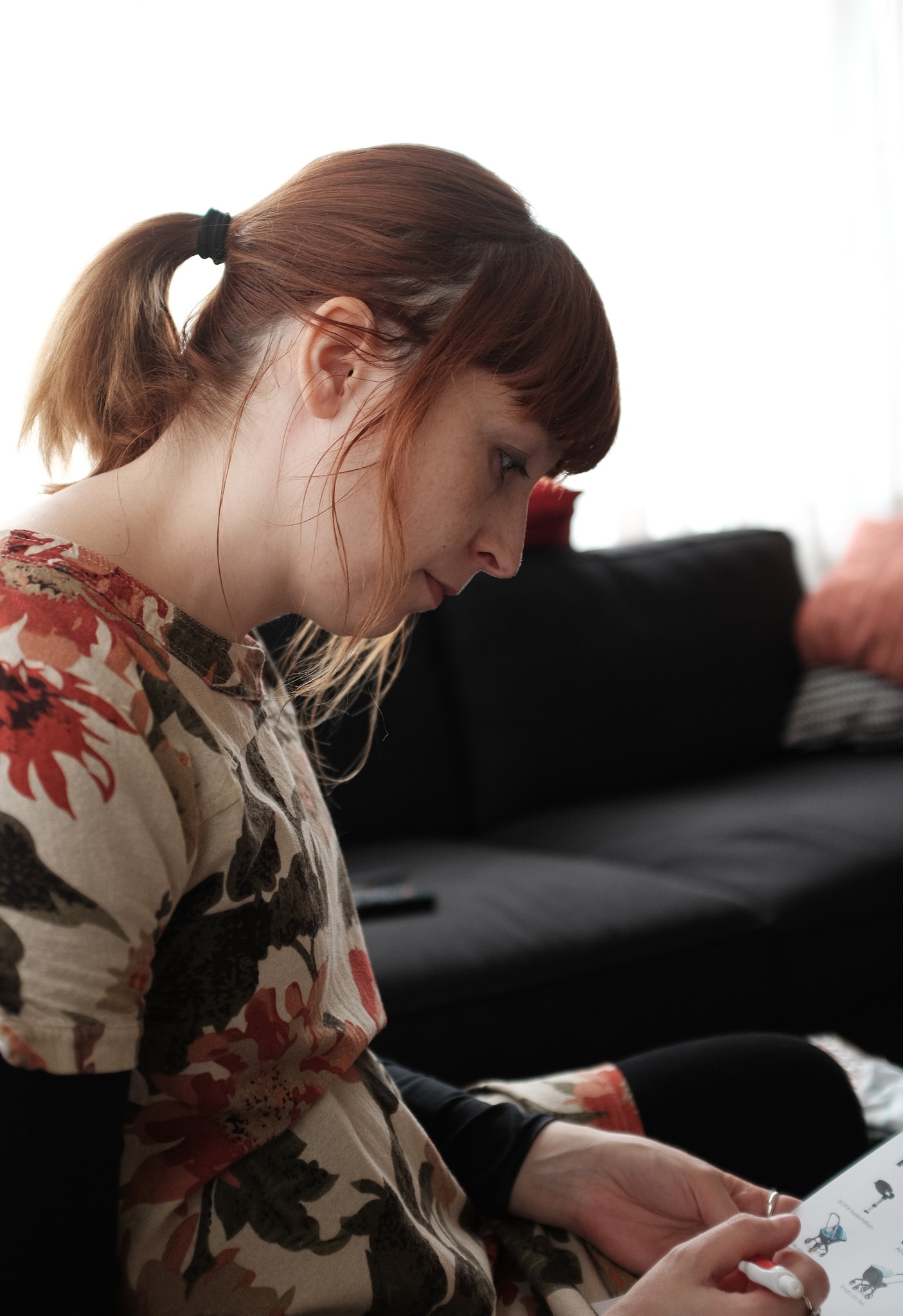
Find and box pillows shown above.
[784,516,902,684]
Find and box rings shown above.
[767,1192,779,1218]
[802,1293,821,1316]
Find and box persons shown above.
[0,146,868,1316]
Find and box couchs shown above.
[261,528,903,1090]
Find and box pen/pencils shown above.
[732,1257,808,1303]
